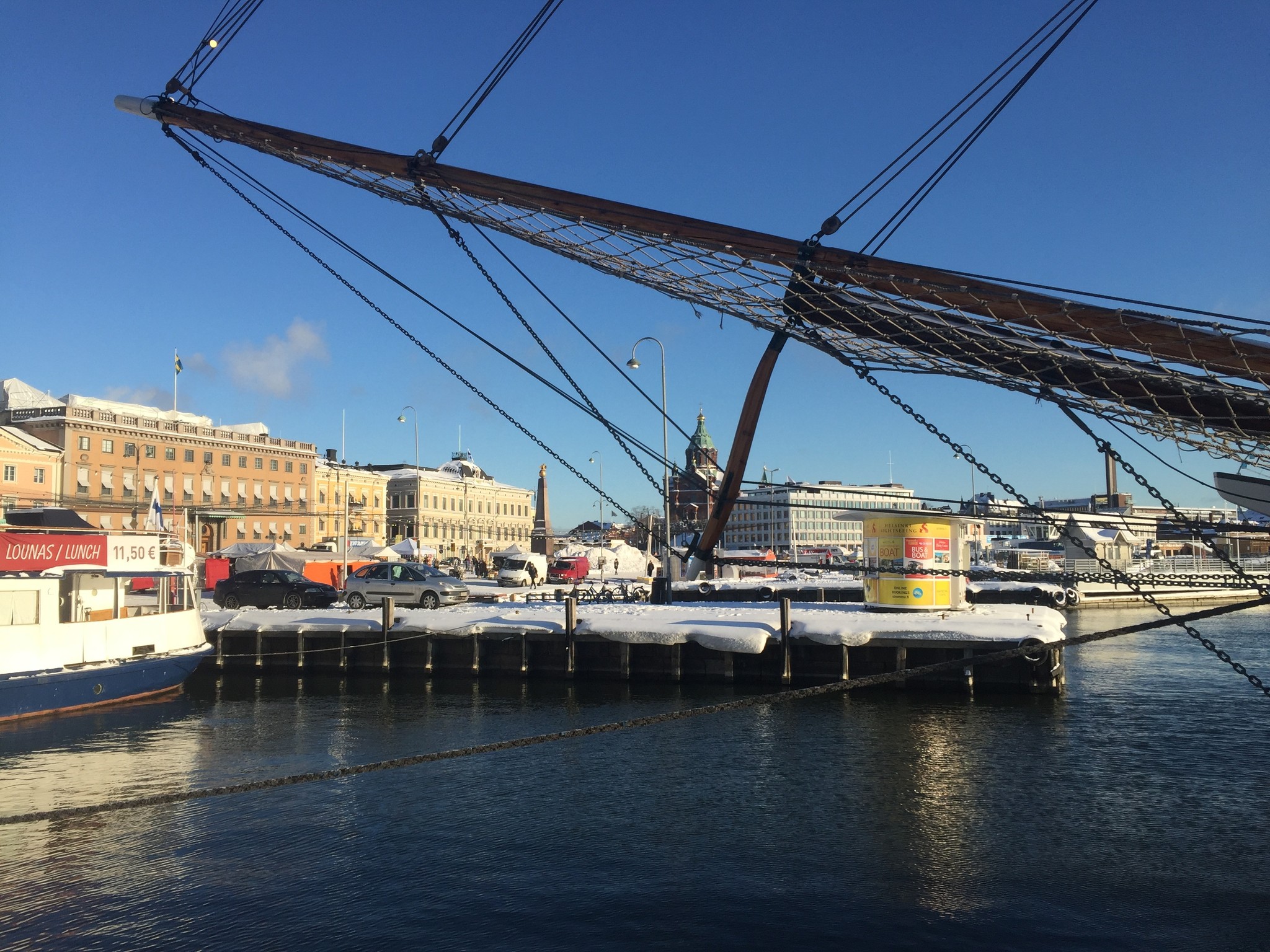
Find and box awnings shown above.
[104,567,195,578]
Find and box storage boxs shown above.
[159,547,182,567]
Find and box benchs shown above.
[88,606,129,622]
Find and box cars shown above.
[213,569,339,610]
[342,562,470,610]
[440,557,461,567]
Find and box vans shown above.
[497,554,548,588]
[547,556,589,586]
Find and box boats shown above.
[0,505,215,724]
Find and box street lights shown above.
[476,540,487,560]
[953,445,978,566]
[442,538,454,557]
[326,464,351,553]
[456,477,477,556]
[763,465,781,553]
[122,438,155,530]
[589,451,609,583]
[525,486,538,522]
[627,337,672,605]
[642,507,656,551]
[398,406,421,563]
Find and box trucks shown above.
[789,545,851,569]
[311,536,375,553]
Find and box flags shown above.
[144,479,166,532]
[611,510,617,517]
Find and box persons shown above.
[465,556,489,578]
[336,564,348,591]
[528,563,538,589]
[979,554,983,560]
[547,560,552,571]
[970,555,974,562]
[647,560,654,577]
[424,554,440,570]
[613,558,619,575]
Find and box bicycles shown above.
[577,580,611,605]
[612,578,647,604]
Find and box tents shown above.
[489,543,531,579]
[208,537,436,564]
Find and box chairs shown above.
[370,569,387,579]
[391,570,398,580]
[400,566,406,579]
[265,573,274,583]
